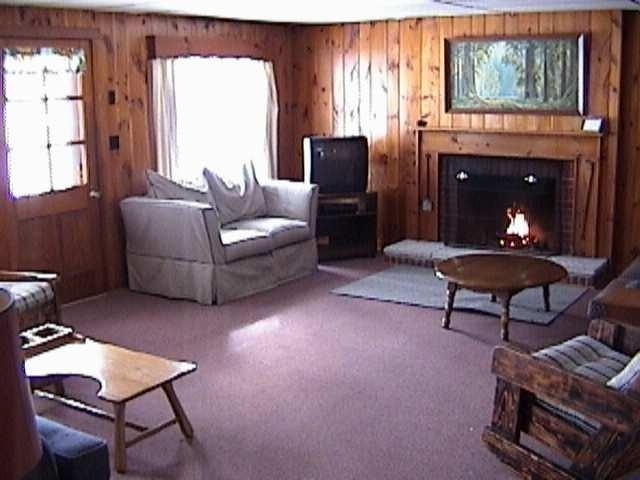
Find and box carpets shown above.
[327,261,591,329]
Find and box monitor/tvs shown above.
[303,137,369,197]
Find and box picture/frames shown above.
[441,31,586,119]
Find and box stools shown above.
[0,268,63,329]
[33,404,110,480]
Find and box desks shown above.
[17,320,201,470]
[588,251,640,327]
[432,249,569,343]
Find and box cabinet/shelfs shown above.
[314,191,379,263]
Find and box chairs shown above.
[482,316,639,478]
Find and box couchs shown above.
[115,173,325,306]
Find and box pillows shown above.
[201,157,271,228]
[143,168,216,225]
